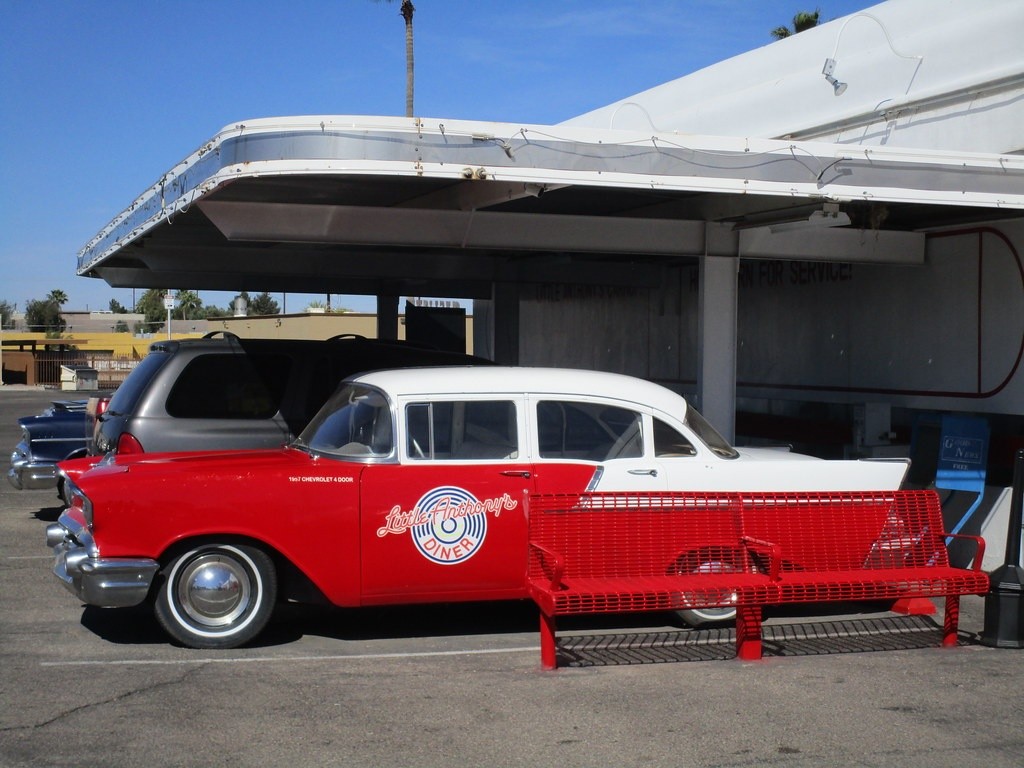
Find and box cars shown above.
[45,364,950,649]
[6,390,117,508]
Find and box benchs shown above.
[526,490,992,672]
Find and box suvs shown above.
[82,330,594,457]
